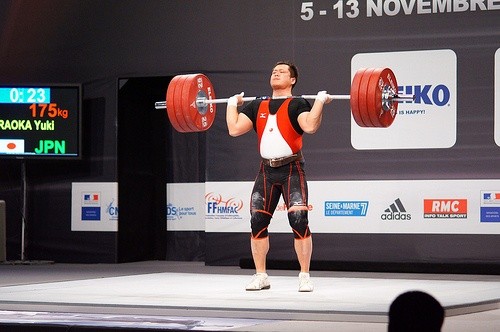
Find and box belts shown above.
[263,154,298,168]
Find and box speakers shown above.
[116,75,207,263]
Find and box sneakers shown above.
[245,274,270,289]
[297,275,314,292]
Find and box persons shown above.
[387,290,445,332]
[226,62,333,292]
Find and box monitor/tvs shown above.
[0,83,83,159]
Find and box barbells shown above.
[153,67,415,134]
